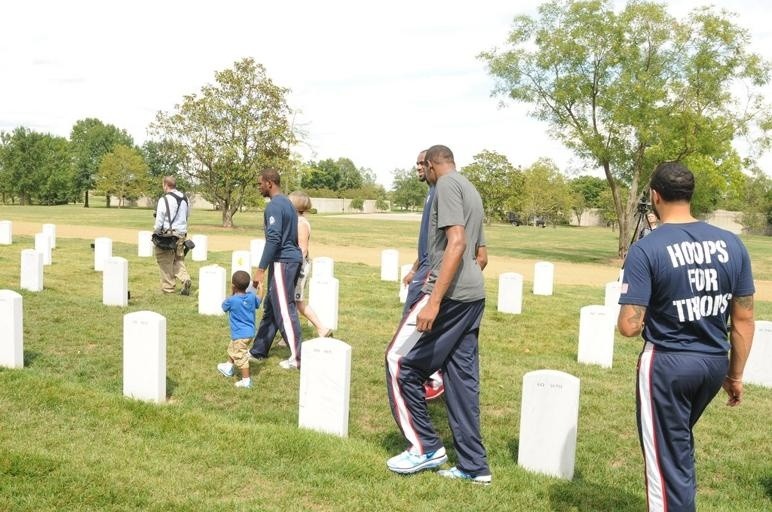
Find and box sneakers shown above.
[217,363,233,376]
[387,446,448,474]
[235,380,250,388]
[438,465,491,486]
[279,359,300,369]
[423,370,444,400]
[248,349,262,361]
[181,279,191,295]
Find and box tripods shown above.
[617,215,653,282]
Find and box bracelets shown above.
[726,375,743,382]
[410,270,416,276]
[258,268,266,274]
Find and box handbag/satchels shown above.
[152,234,178,250]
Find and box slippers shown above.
[324,329,334,337]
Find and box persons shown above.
[277,191,335,347]
[216,270,265,388]
[385,143,493,485]
[402,148,446,402]
[150,176,192,296]
[616,160,756,512]
[251,167,304,368]
[637,212,657,242]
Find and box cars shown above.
[509,211,547,229]
